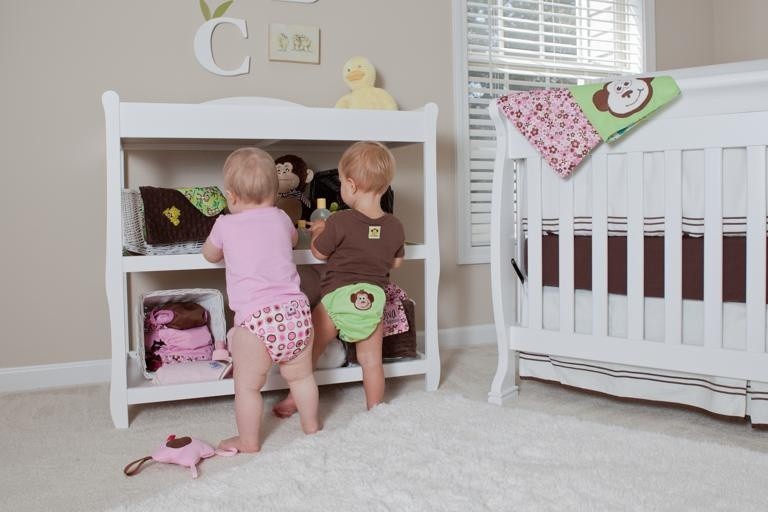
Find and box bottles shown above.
[296,198,331,249]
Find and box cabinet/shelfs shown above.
[100,89,440,429]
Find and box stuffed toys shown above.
[271,154,314,232]
[153,433,238,479]
[332,55,400,111]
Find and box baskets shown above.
[135,287,228,381]
[121,187,229,255]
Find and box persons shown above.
[200,146,327,455]
[268,139,409,418]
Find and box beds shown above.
[486,58,766,408]
